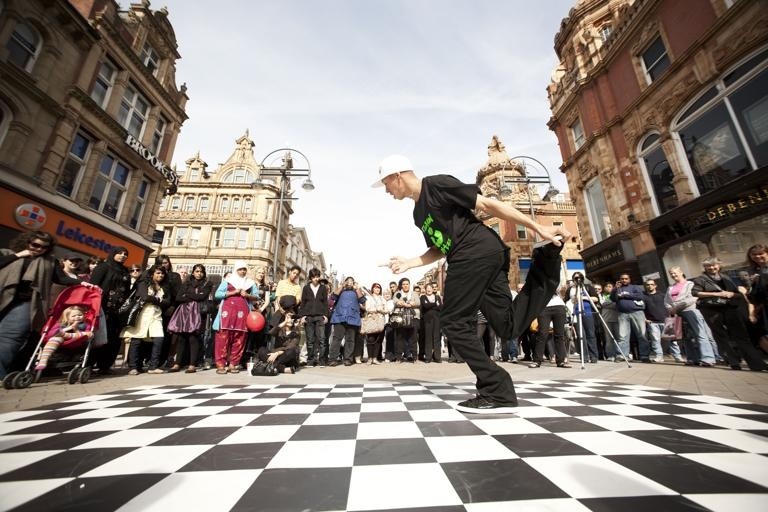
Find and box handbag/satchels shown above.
[693,276,727,309]
[114,288,146,327]
[661,310,683,341]
[388,307,404,328]
[359,311,384,335]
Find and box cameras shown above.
[575,276,584,282]
[346,282,353,287]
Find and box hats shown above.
[64,254,84,261]
[370,160,413,188]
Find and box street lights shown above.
[497,155,559,245]
[252,148,316,289]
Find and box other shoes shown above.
[615,356,714,367]
[356,359,443,365]
[490,356,573,368]
[305,358,352,367]
[216,364,246,374]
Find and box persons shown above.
[370,154,570,415]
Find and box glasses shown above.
[29,241,49,250]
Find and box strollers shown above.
[3,282,106,391]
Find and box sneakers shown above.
[456,395,518,415]
[98,355,215,375]
[532,235,565,256]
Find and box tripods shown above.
[566,282,632,369]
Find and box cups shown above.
[246,363,255,376]
